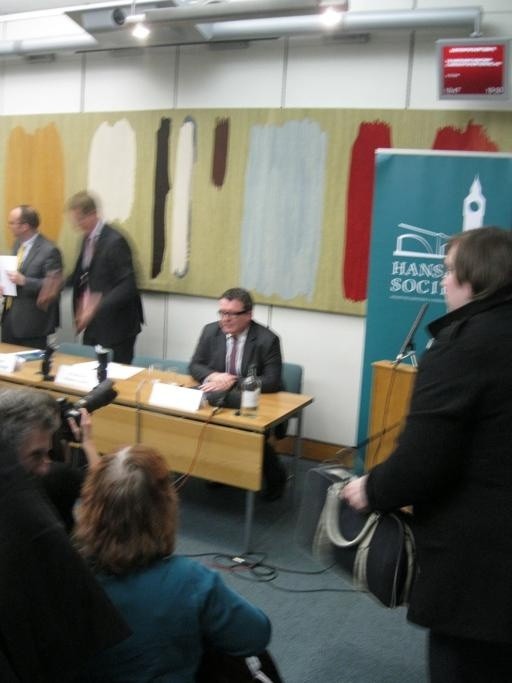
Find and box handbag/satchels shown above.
[295,467,419,610]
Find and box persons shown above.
[1,201,63,351]
[1,388,100,531]
[336,223,511,679]
[36,186,148,370]
[185,285,296,515]
[2,438,137,681]
[68,443,274,682]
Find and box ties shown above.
[230,334,237,375]
[5,244,24,309]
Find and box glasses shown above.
[218,310,250,317]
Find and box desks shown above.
[0,343,313,555]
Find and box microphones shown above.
[395,302,430,362]
[214,379,239,406]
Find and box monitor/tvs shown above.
[435,37,512,101]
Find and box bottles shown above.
[238,362,263,418]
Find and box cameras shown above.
[56,378,120,439]
